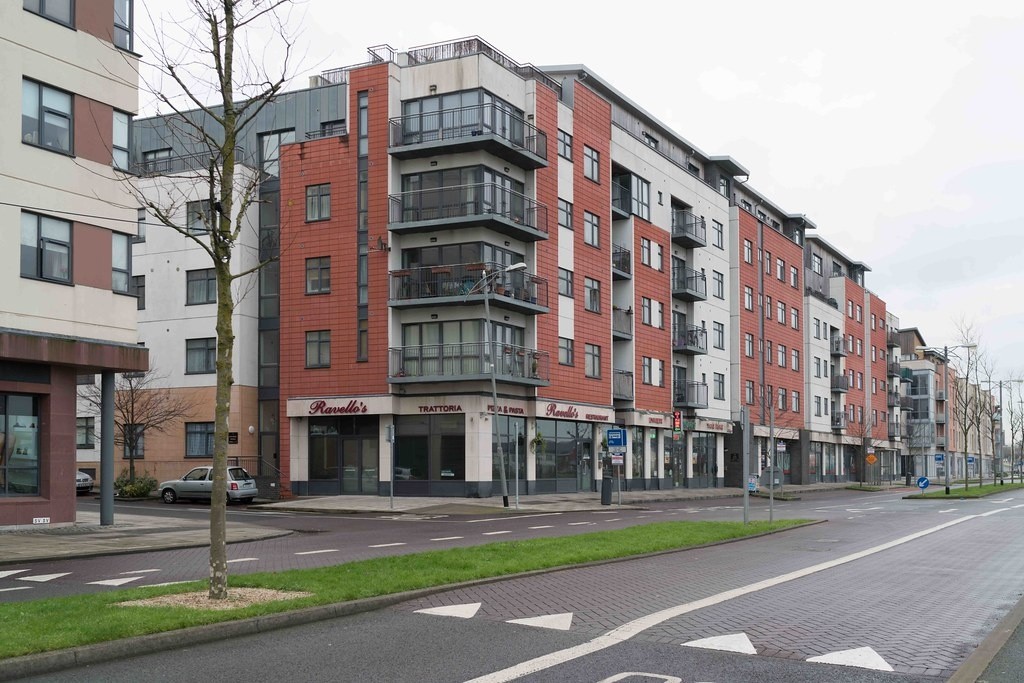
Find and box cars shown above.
[157,465,259,507]
[76,471,94,496]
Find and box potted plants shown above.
[528,431,548,456]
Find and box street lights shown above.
[931,344,979,495]
[987,378,1024,485]
[462,262,529,507]
